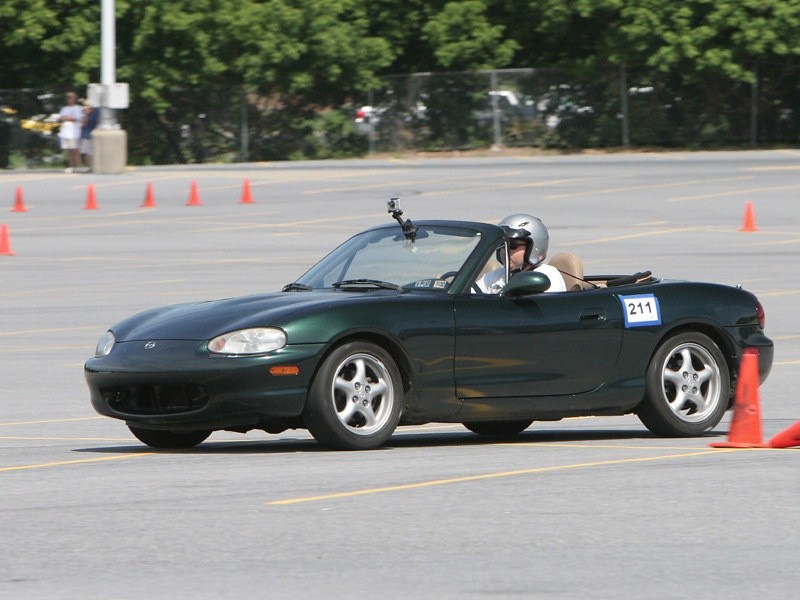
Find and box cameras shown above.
[388,198,400,212]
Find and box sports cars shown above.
[85,198,775,453]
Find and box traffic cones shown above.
[13,186,30,212]
[184,181,202,205]
[740,202,757,232]
[768,420,800,448]
[1,225,14,254]
[709,353,767,449]
[239,178,253,205]
[82,185,99,209]
[141,184,156,206]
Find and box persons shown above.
[57,91,100,171]
[471,214,567,293]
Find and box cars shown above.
[357,87,662,150]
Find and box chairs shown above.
[547,252,584,292]
[476,257,502,281]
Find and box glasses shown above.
[502,241,527,250]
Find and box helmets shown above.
[496,214,549,266]
